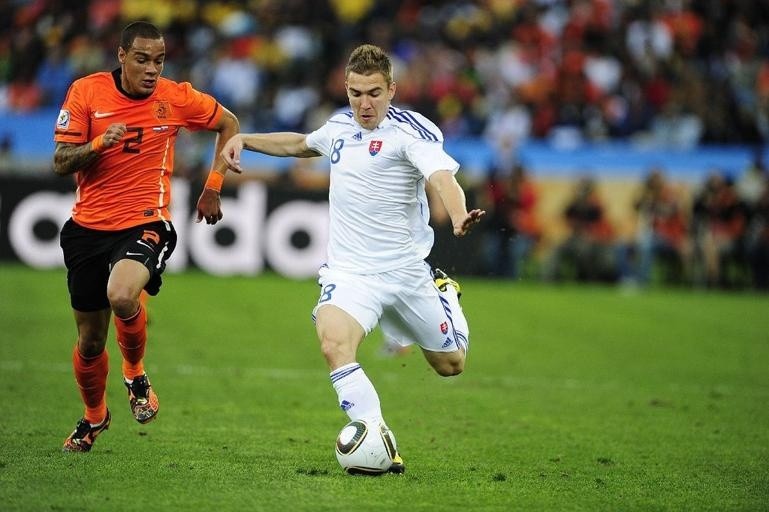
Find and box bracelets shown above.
[204,170,223,193]
[91,135,105,153]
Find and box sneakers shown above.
[122,370,162,426]
[388,452,405,474]
[432,267,464,299]
[62,406,112,453]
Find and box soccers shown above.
[334,416,396,476]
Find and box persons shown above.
[52,23,241,453]
[221,44,487,473]
